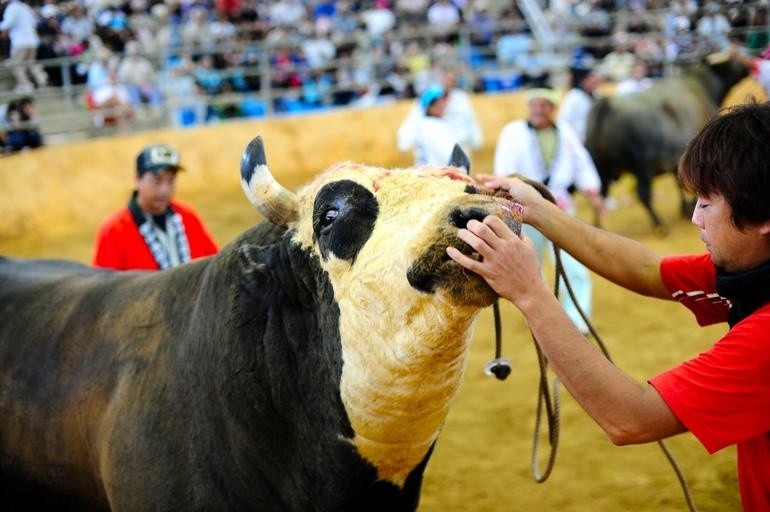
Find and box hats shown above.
[136,144,184,173]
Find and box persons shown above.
[445,91,767,509]
[395,82,472,175]
[493,77,609,360]
[92,143,219,272]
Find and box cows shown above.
[582,38,753,240]
[0,134,525,512]
